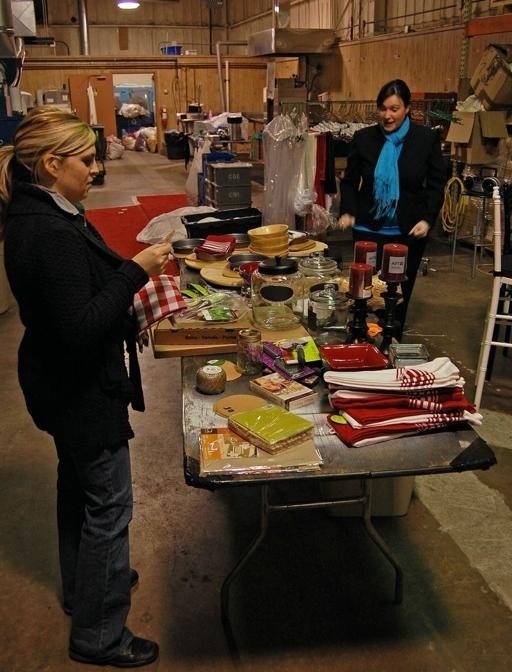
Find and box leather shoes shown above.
[63,568,160,667]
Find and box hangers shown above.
[265,100,368,146]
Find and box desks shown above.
[175,256,498,661]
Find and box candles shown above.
[349,239,408,301]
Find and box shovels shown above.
[263,339,308,376]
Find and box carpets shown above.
[85,194,193,272]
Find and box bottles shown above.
[249,259,306,330]
[307,284,354,347]
[236,329,263,376]
[300,255,343,321]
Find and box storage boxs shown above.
[441,42,510,253]
[196,152,253,210]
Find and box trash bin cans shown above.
[164,132,187,160]
[319,474,414,518]
[116,112,154,139]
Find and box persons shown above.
[0,107,173,667]
[337,79,445,341]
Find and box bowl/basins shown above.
[227,233,250,249]
[248,223,289,258]
[226,254,269,272]
[172,238,205,255]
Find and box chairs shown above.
[473,176,510,421]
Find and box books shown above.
[196,372,320,477]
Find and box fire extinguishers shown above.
[161,108,168,129]
